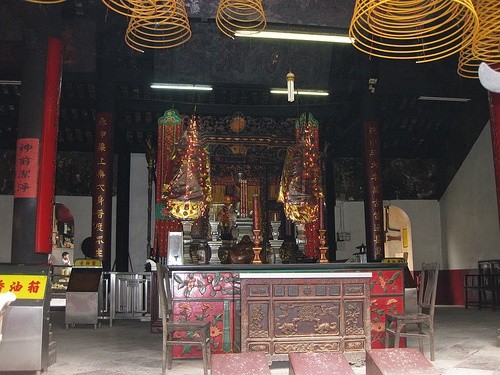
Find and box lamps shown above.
[230,28,356,44]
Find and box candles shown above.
[318,195,325,231]
[253,194,261,230]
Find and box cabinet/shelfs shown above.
[477,259,500,312]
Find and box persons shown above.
[292,125,319,202]
[218,203,237,221]
[167,123,205,198]
[60,252,70,269]
[228,183,241,210]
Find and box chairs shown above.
[383,263,438,362]
[155,262,212,375]
